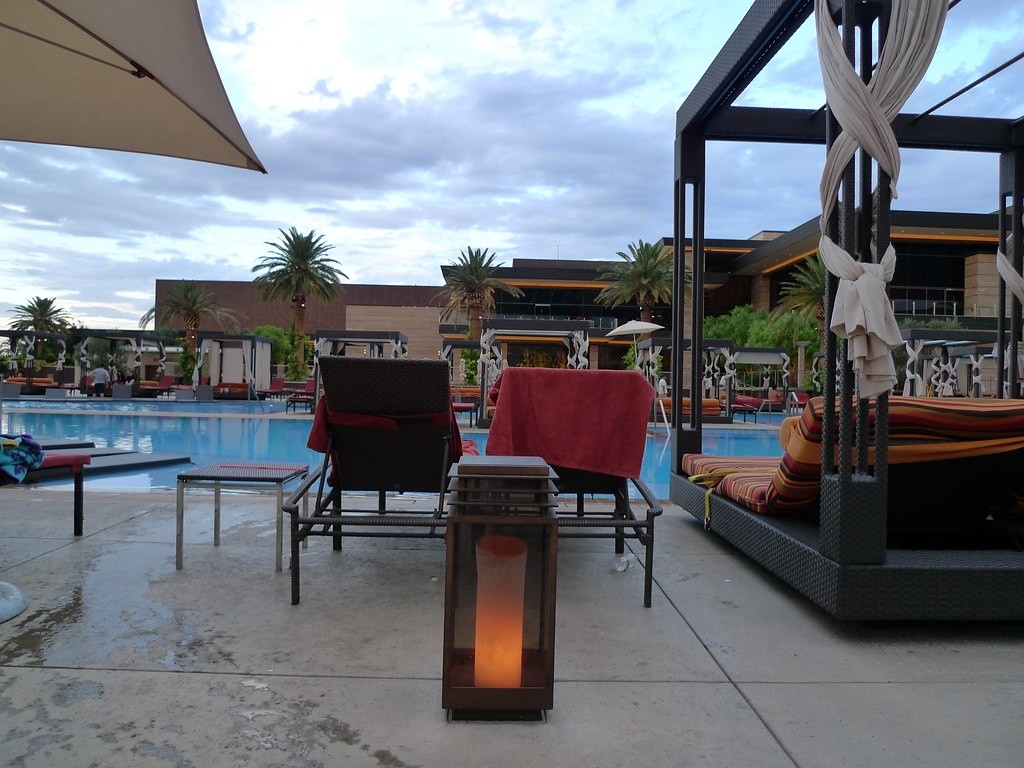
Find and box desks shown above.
[176,464,308,572]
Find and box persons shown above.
[92,364,109,398]
[658,374,668,398]
[769,385,782,400]
[116,370,125,385]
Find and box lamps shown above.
[473,536,527,690]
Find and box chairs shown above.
[0,455,91,536]
[140,375,173,399]
[258,377,315,414]
[281,356,479,604]
[32,376,111,397]
[200,376,210,385]
[486,368,664,607]
[452,402,478,428]
[792,392,809,410]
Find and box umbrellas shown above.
[605,320,666,372]
[0,0,267,175]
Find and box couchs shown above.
[656,397,720,416]
[682,397,1024,519]
[451,387,481,406]
[719,394,782,411]
[7,377,52,395]
[131,380,159,399]
[214,382,255,400]
